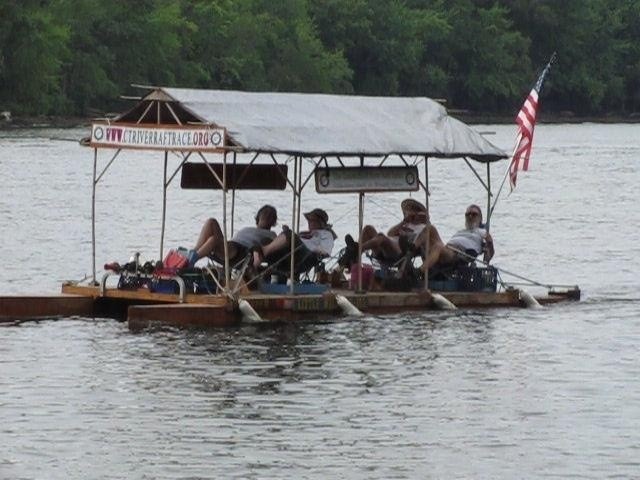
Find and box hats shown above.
[304,210,328,225]
[401,200,427,222]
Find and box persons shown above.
[407,204,495,274]
[178,204,279,273]
[344,198,444,262]
[246,208,337,276]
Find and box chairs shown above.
[208,237,491,295]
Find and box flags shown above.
[506,58,556,195]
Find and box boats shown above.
[0,283,580,327]
